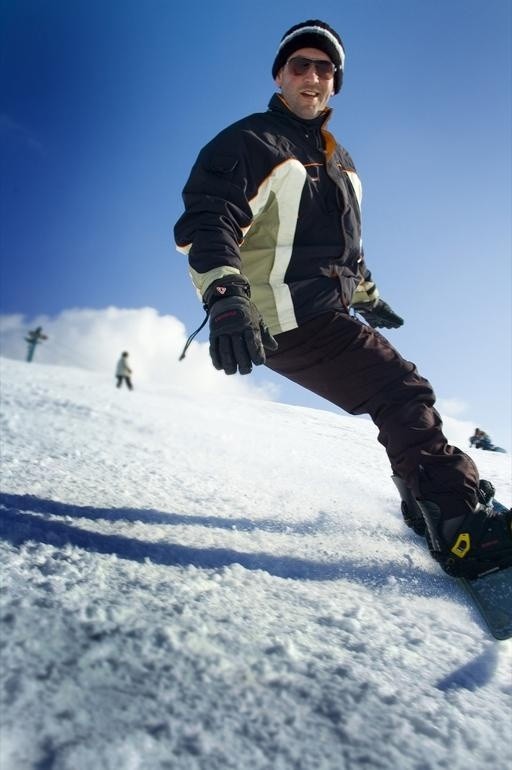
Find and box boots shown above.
[389,473,495,538]
[415,495,511,581]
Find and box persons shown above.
[114,351,134,389]
[473,426,492,451]
[174,18,512,582]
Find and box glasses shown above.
[284,55,338,82]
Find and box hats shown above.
[271,17,346,95]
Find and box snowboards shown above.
[459,484,511,644]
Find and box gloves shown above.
[351,296,405,331]
[200,273,279,378]
